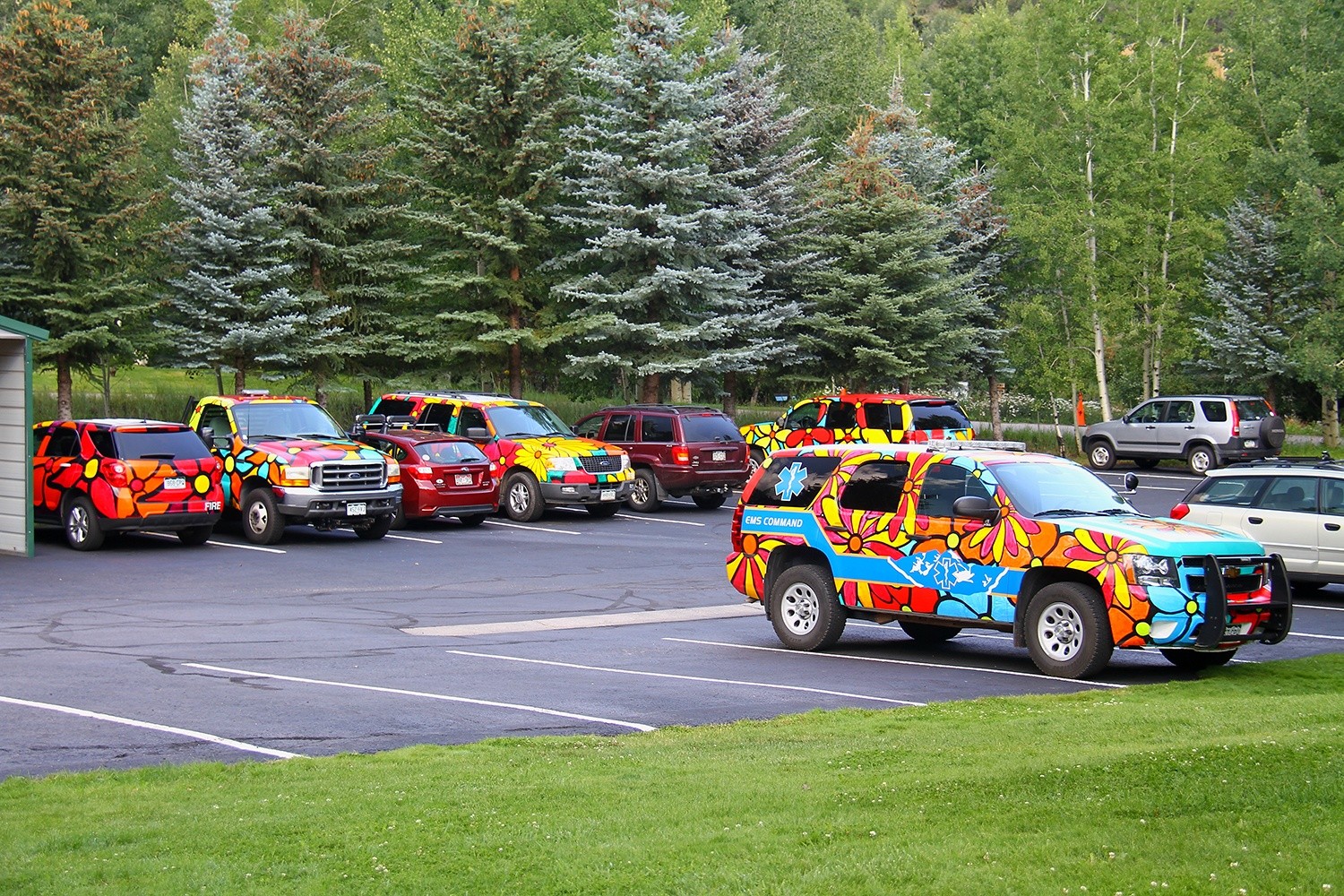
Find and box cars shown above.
[343,413,501,527]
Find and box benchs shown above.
[1204,406,1225,421]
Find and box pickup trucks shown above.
[177,388,403,545]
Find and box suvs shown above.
[569,401,753,513]
[1082,394,1287,476]
[365,389,636,522]
[1167,455,1344,599]
[721,440,1296,680]
[737,390,980,491]
[31,418,226,551]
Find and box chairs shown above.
[441,446,457,459]
[934,480,963,515]
[209,417,233,446]
[1265,487,1344,515]
[1169,406,1182,422]
